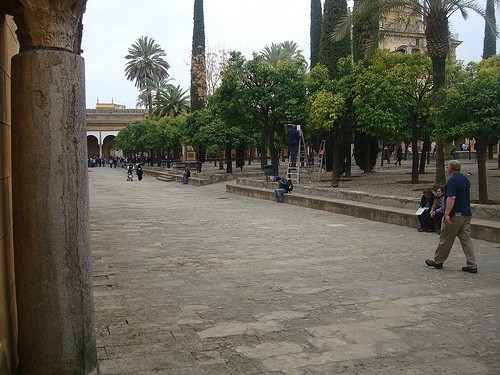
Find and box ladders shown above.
[311,140,328,182]
[285,128,311,185]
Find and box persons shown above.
[182,167,190,183]
[424,160,478,273]
[88,153,143,180]
[417,184,444,234]
[275,177,289,202]
[394,143,471,167]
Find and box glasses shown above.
[446,166,449,169]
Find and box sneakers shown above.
[462,267,477,273]
[425,260,443,269]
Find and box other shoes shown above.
[427,229,434,232]
[280,197,284,203]
[418,228,426,232]
[277,198,279,203]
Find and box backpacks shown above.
[288,180,293,190]
